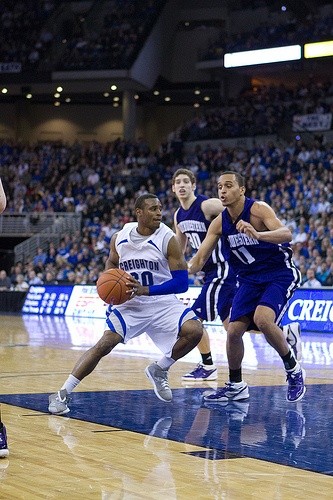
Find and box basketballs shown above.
[97,268,132,304]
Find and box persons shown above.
[172,169,302,380]
[48,194,203,415]
[181,386,306,500]
[48,414,176,500]
[0,79,333,293]
[187,171,307,403]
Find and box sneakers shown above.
[48,389,71,415]
[203,380,250,402]
[144,361,172,402]
[180,362,217,381]
[284,363,306,402]
[284,321,302,361]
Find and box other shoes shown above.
[0,421,9,458]
